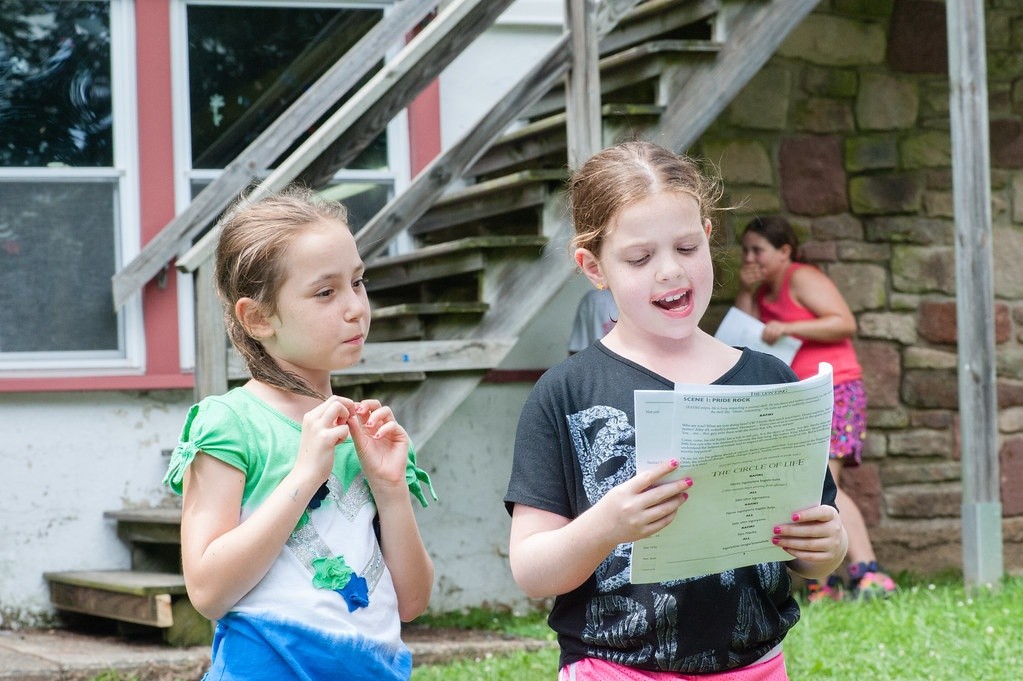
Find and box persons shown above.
[165,188,432,681]
[507,141,849,680]
[566,280,621,356]
[736,218,900,605]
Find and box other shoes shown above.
[807,576,845,603]
[847,560,897,599]
